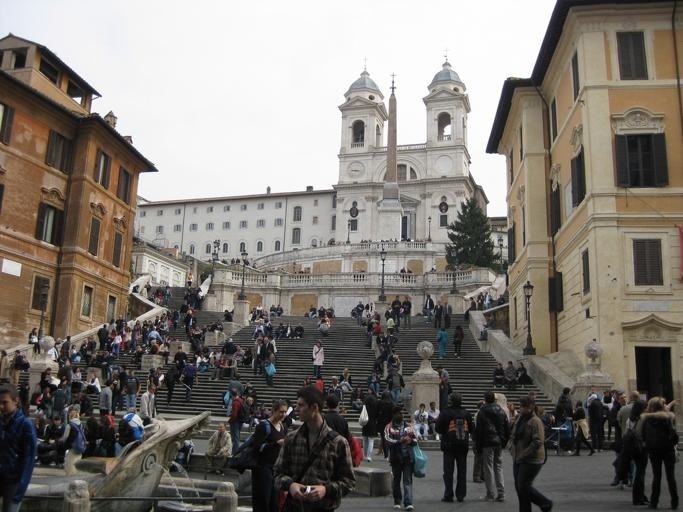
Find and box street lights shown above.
[346,219,350,243]
[237,250,248,300]
[497,236,504,274]
[427,216,431,242]
[32,281,50,354]
[378,248,387,301]
[211,240,219,269]
[523,280,536,355]
[207,250,217,294]
[450,248,458,294]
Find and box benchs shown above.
[352,466,392,497]
[191,451,242,474]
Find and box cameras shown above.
[302,486,311,496]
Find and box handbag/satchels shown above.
[279,490,289,512]
[413,443,428,478]
[264,360,277,377]
[359,405,369,426]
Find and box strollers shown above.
[544,417,572,458]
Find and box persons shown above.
[245,382,258,417]
[413,402,441,442]
[131,278,200,310]
[383,405,418,511]
[250,397,288,511]
[437,366,451,411]
[532,383,680,489]
[624,397,681,505]
[433,392,477,501]
[474,390,511,502]
[273,384,356,511]
[465,290,505,340]
[493,360,530,391]
[313,341,325,378]
[641,396,679,511]
[245,304,303,385]
[0,381,38,511]
[423,294,463,360]
[306,304,335,336]
[346,294,412,460]
[507,395,553,512]
[328,369,352,415]
[1,302,244,468]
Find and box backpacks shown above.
[68,421,87,454]
[623,420,641,450]
[232,398,250,424]
[441,407,469,441]
[228,419,285,474]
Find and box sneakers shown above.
[405,505,414,511]
[392,504,401,509]
[441,497,505,502]
